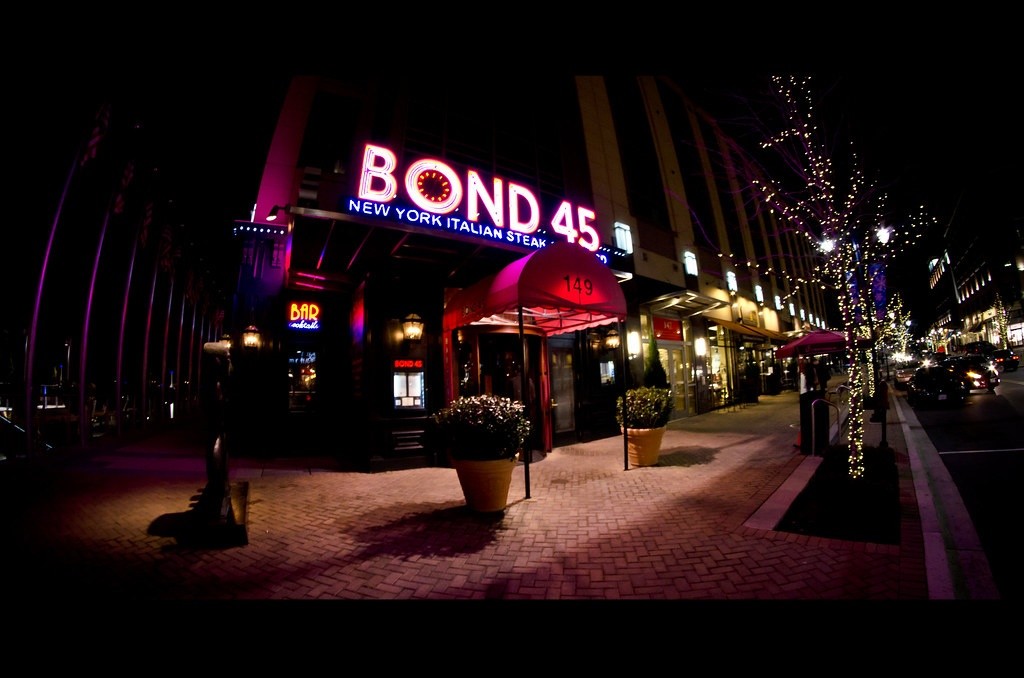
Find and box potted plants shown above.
[616,384,674,466]
[418,395,530,512]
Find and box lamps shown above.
[266,204,291,221]
[602,326,620,356]
[399,310,424,343]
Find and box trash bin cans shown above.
[800,391,830,453]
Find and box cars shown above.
[906,366,966,410]
[939,355,1001,394]
[893,341,1019,390]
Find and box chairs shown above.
[714,387,742,413]
[828,382,848,404]
